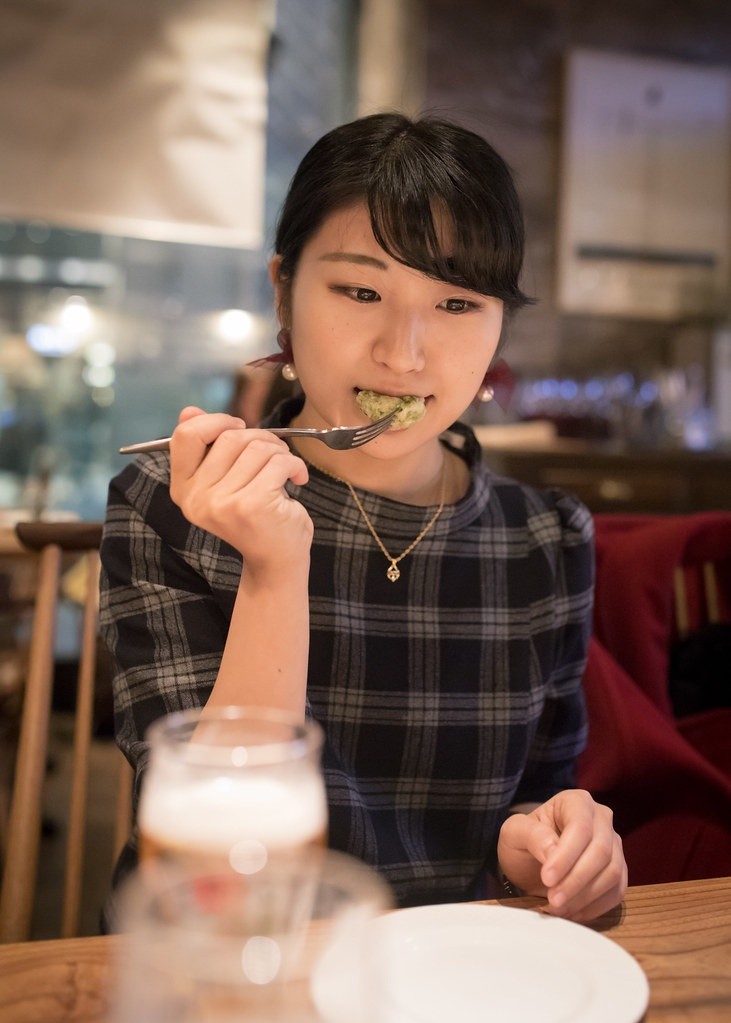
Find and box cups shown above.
[106,849,386,1023]
[136,707,329,948]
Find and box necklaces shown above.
[306,454,445,580]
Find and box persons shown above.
[97,114,628,923]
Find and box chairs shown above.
[0,521,133,942]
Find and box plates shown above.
[310,902,649,1023]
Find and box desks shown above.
[0,876,731,1023]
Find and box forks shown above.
[119,407,402,453]
